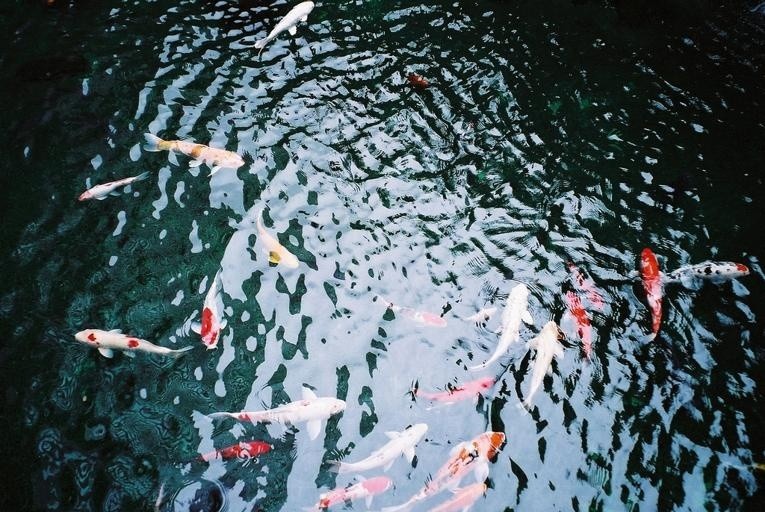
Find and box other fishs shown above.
[253,0,315,57]
